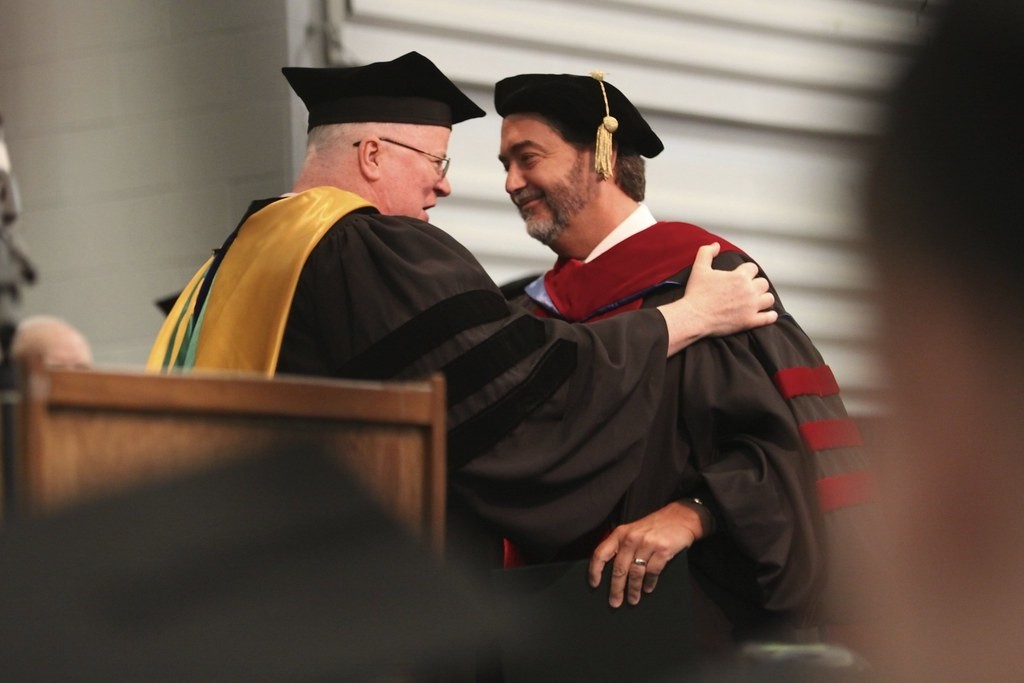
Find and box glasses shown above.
[352,138,452,180]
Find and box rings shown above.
[632,558,648,566]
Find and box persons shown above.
[1,312,93,410]
[154,51,781,581]
[489,71,878,681]
[844,0,1023,683]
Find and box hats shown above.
[282,52,486,131]
[495,75,664,180]
